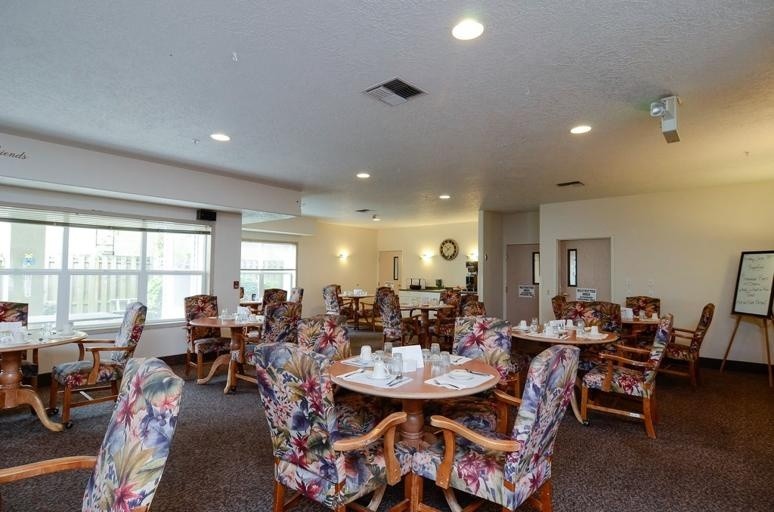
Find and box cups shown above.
[14,330,28,342]
[344,288,368,295]
[624,308,658,321]
[221,306,256,321]
[518,317,599,336]
[63,321,73,335]
[252,293,257,301]
[360,342,450,379]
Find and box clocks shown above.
[441,240,457,260]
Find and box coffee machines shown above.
[465,261,477,294]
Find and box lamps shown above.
[650,96,680,143]
